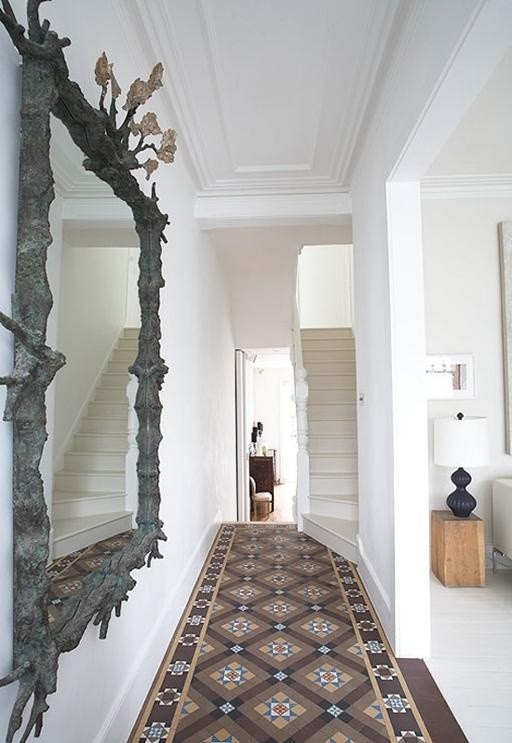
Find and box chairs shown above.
[249,476,272,520]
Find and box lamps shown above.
[433,412,488,517]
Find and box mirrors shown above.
[0,0,180,743]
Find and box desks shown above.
[432,510,486,588]
[249,450,275,512]
